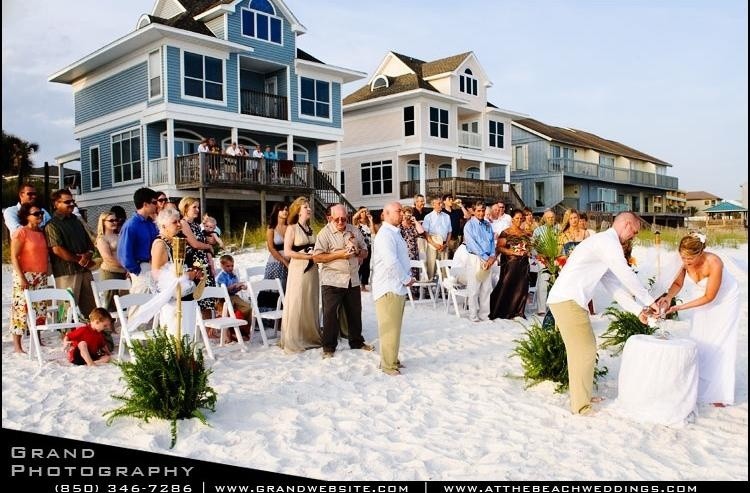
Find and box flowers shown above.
[513,240,532,257]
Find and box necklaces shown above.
[693,258,706,276]
[567,226,580,237]
[296,221,313,236]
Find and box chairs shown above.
[398,257,555,321]
[21,258,289,370]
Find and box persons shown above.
[162,202,178,210]
[44,188,96,338]
[197,140,210,180]
[254,200,291,330]
[528,271,538,294]
[533,208,563,318]
[93,211,131,323]
[198,212,224,277]
[234,144,250,183]
[519,207,539,236]
[555,208,591,268]
[207,138,227,182]
[351,206,376,294]
[156,191,167,213]
[225,141,242,183]
[217,255,253,342]
[577,212,593,237]
[3,183,52,240]
[310,202,378,360]
[484,206,501,248]
[413,193,429,225]
[149,206,197,330]
[397,205,425,300]
[655,233,741,410]
[545,210,661,420]
[422,195,453,300]
[61,307,115,366]
[110,206,127,234]
[495,199,513,228]
[441,194,471,260]
[490,209,533,322]
[262,143,278,184]
[9,202,50,355]
[250,143,265,183]
[368,201,416,377]
[462,200,496,323]
[178,196,221,339]
[374,208,385,234]
[277,196,324,352]
[116,186,160,333]
[491,203,510,235]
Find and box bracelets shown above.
[353,247,362,257]
[444,243,449,247]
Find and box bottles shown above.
[642,305,654,318]
[659,302,668,320]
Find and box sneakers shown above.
[361,345,375,352]
[382,368,400,376]
[397,361,404,367]
[322,352,333,359]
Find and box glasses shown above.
[157,198,168,202]
[103,218,118,222]
[152,201,157,204]
[334,217,346,223]
[59,199,75,204]
[30,211,44,216]
[23,191,36,196]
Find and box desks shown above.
[618,333,700,430]
